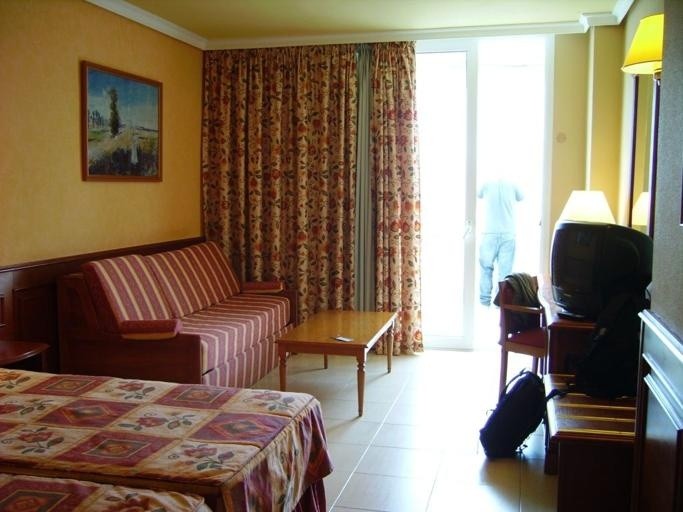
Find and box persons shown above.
[478,175,524,306]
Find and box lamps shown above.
[620,12,664,87]
[556,190,615,224]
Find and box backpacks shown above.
[577,296,636,399]
[480,368,572,459]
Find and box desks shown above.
[539,374,636,512]
[547,316,598,374]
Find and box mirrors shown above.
[629,74,661,240]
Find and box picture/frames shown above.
[79,59,164,183]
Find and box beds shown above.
[1,472,212,512]
[0,366,334,512]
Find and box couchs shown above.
[54,240,300,388]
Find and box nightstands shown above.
[0,337,50,370]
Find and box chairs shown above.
[499,275,551,401]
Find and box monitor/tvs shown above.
[549,219,654,320]
